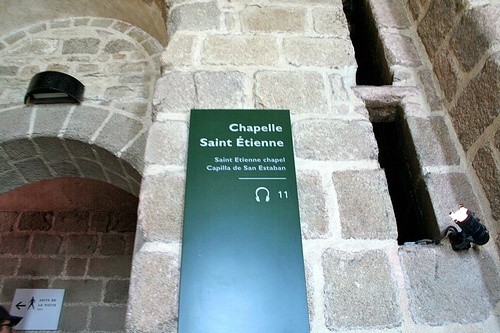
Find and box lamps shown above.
[446,204,491,251]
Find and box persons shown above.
[0,302,26,333]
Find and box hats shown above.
[0,304,23,327]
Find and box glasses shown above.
[0,321,14,328]
